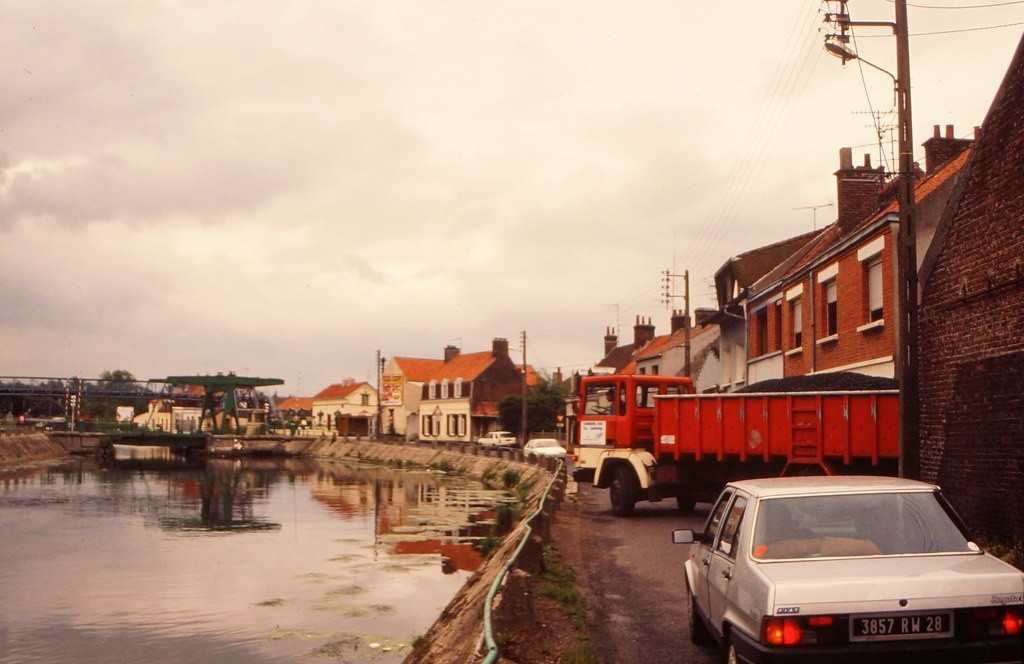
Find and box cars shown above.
[669,475,1023,664]
[524,439,566,462]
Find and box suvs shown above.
[476,430,517,449]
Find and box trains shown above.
[16,419,139,435]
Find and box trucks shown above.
[566,373,900,516]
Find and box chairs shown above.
[852,515,894,555]
[764,504,796,549]
